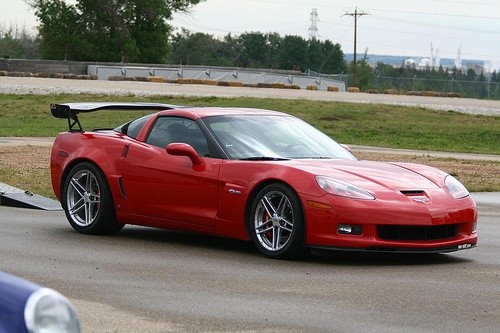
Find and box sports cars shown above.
[50,101,478,257]
